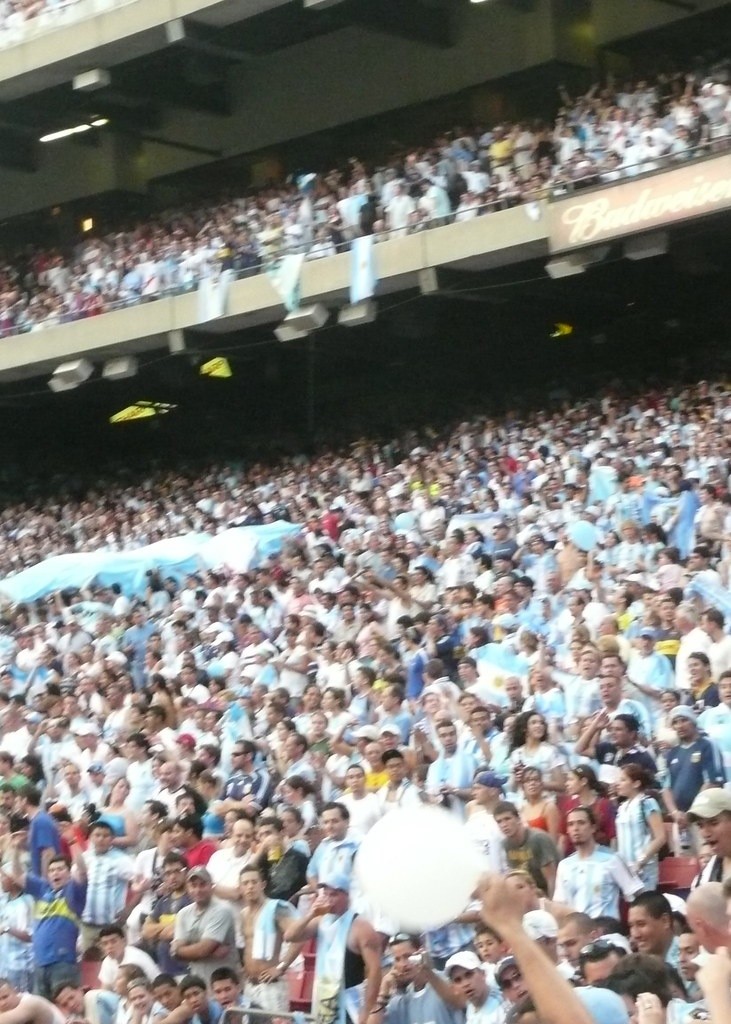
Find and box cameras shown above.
[47,718,60,727]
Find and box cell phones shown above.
[689,1008,710,1021]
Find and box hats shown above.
[316,874,350,893]
[666,705,697,731]
[684,788,731,823]
[87,763,106,775]
[443,950,481,977]
[522,908,559,941]
[493,955,516,985]
[185,864,211,884]
[104,651,128,665]
[23,711,41,724]
[204,621,235,647]
[473,768,506,788]
[349,724,408,761]
[176,733,196,748]
[2,860,27,888]
[73,721,105,737]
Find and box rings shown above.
[644,1004,652,1011]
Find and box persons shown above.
[0,61,731,1024]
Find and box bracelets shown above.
[3,926,11,933]
[276,961,289,973]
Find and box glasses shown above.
[571,766,584,776]
[388,933,412,944]
[579,939,609,954]
[499,972,521,992]
[231,751,250,758]
